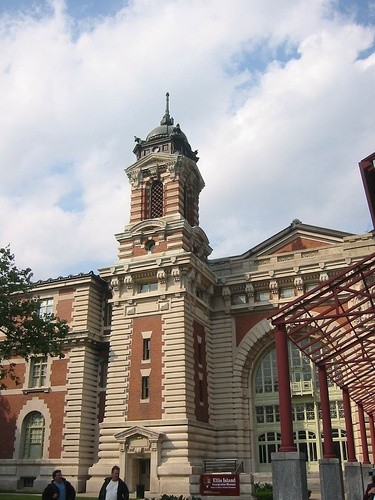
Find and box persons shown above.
[363,471,375,500]
[42,470,76,500]
[98,466,129,500]
